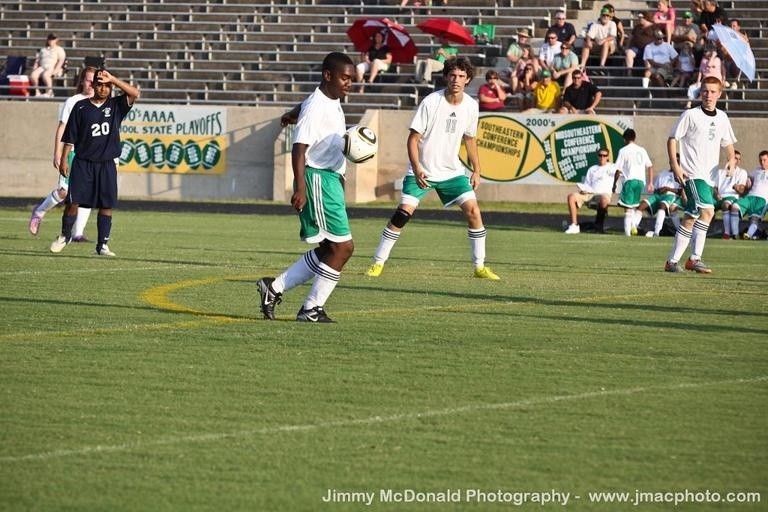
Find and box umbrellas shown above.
[346,17,419,64]
[416,18,476,45]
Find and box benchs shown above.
[1,0,766,128]
[585,197,768,235]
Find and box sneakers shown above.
[625,225,661,237]
[72,236,94,243]
[564,224,580,234]
[256,276,283,320]
[296,304,337,324]
[665,260,685,273]
[28,206,42,234]
[722,233,749,240]
[471,266,500,281]
[49,234,72,253]
[685,256,712,274]
[368,262,384,279]
[95,244,115,256]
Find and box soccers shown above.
[339,126,377,163]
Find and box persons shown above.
[664,76,738,274]
[410,37,459,83]
[29,33,66,96]
[564,128,768,240]
[478,1,750,115]
[50,69,140,257]
[29,66,97,243]
[356,32,393,93]
[257,53,354,324]
[364,55,500,280]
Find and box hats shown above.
[516,28,529,37]
[652,29,665,39]
[541,69,551,77]
[682,11,692,18]
[600,8,610,15]
[554,12,565,18]
